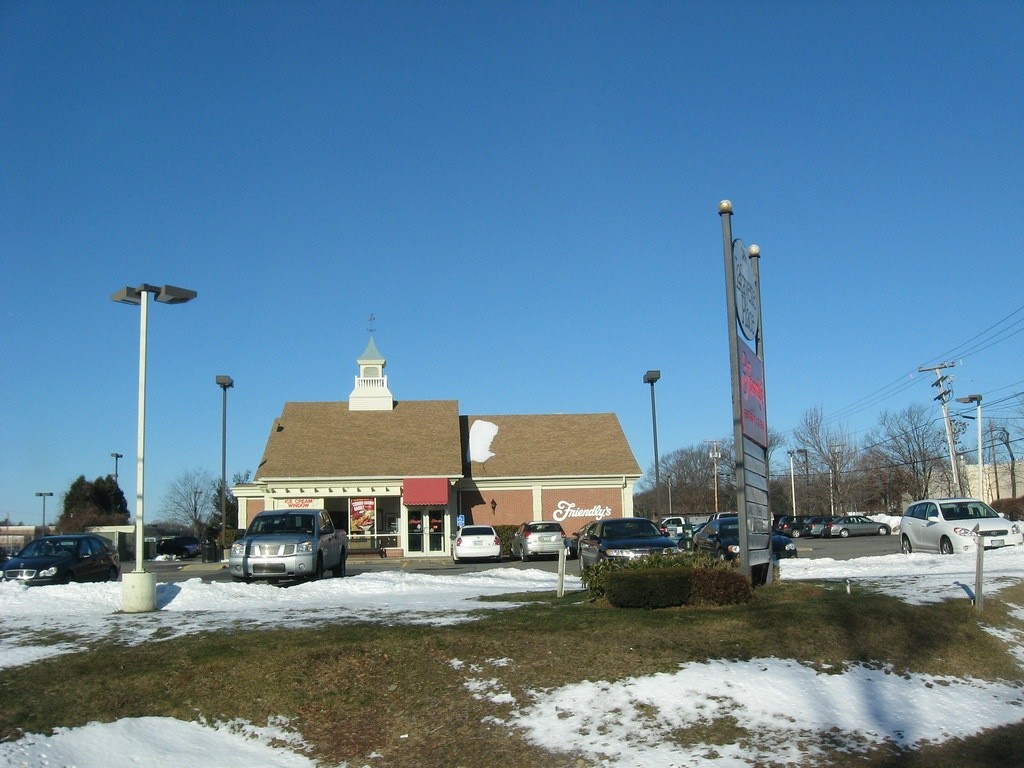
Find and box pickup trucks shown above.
[229,509,349,583]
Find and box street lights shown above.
[666,474,673,524]
[35,492,54,536]
[110,453,123,484]
[214,375,235,549]
[954,394,985,518]
[787,449,796,522]
[643,370,662,528]
[111,282,197,572]
[797,448,811,518]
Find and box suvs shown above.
[156,535,201,560]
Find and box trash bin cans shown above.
[201,540,217,563]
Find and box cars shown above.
[0,546,24,564]
[899,497,1024,554]
[449,524,502,564]
[579,517,685,571]
[802,514,842,538]
[692,515,797,564]
[824,515,891,538]
[509,521,572,563]
[0,533,120,587]
[566,510,819,558]
